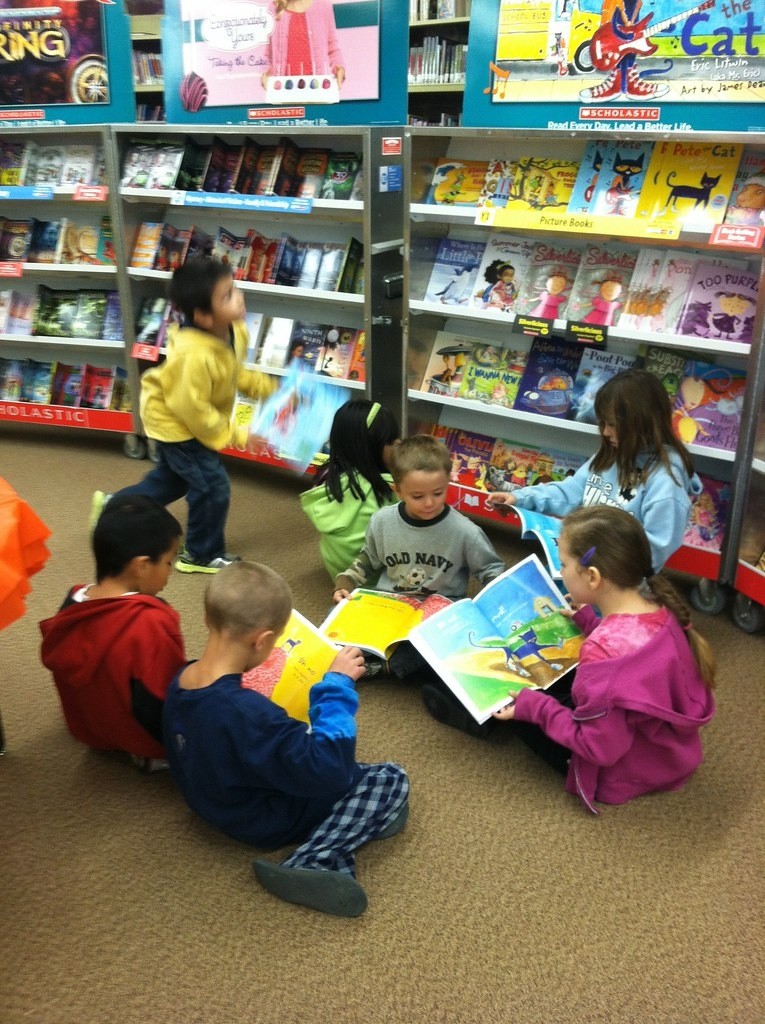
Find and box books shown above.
[317,586,455,660]
[240,609,344,736]
[404,552,588,726]
[1,132,764,582]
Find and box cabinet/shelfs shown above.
[0,0,765,633]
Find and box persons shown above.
[485,367,704,622]
[421,503,717,819]
[158,562,411,918]
[297,397,406,588]
[260,0,346,90]
[37,491,188,773]
[329,435,506,689]
[90,259,278,574]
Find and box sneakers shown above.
[173,541,241,572]
[87,491,114,550]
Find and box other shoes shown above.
[420,683,485,737]
[356,652,387,681]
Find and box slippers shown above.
[250,859,368,918]
